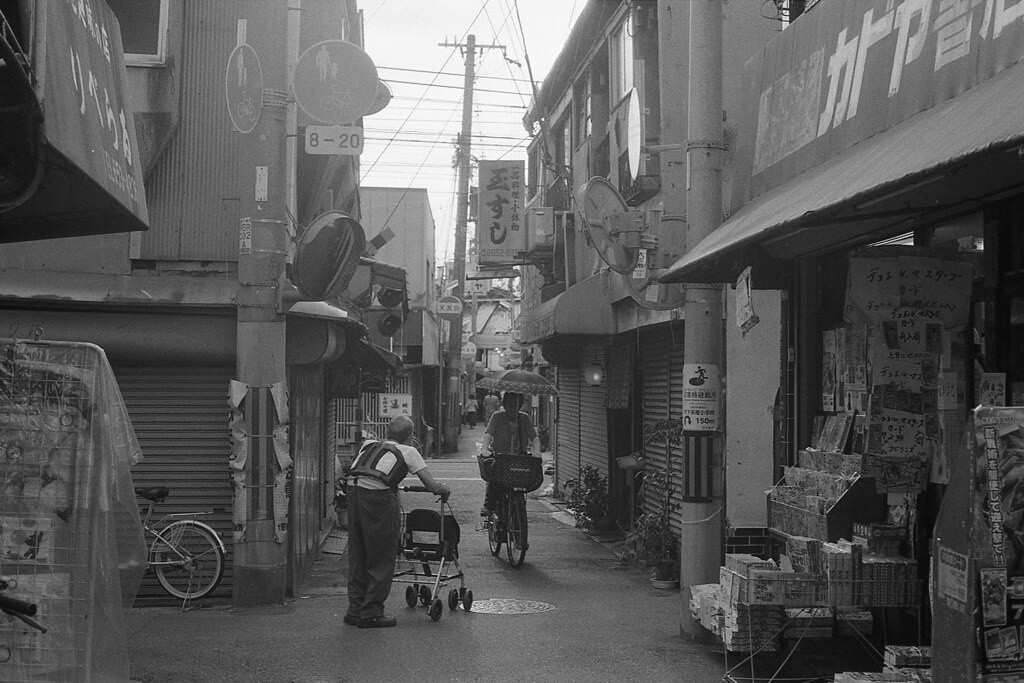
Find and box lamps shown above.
[584,346,607,388]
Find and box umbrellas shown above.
[471,369,562,450]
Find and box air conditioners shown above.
[525,207,553,258]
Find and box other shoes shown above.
[343,612,360,626]
[357,616,397,628]
[480,506,492,516]
[514,540,530,550]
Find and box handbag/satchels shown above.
[492,455,544,493]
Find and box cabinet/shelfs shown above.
[0,338,112,683]
[720,565,923,683]
[765,452,865,546]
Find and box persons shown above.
[344,417,451,627]
[465,388,502,429]
[887,327,897,344]
[927,330,940,350]
[480,392,542,550]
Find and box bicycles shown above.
[478,452,541,568]
[362,414,424,457]
[92,485,228,613]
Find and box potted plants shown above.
[623,419,681,588]
[332,479,349,529]
[561,464,617,530]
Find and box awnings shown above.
[659,60,1024,289]
[361,339,406,384]
[527,271,616,343]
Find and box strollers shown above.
[399,486,474,621]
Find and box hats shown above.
[498,392,525,407]
